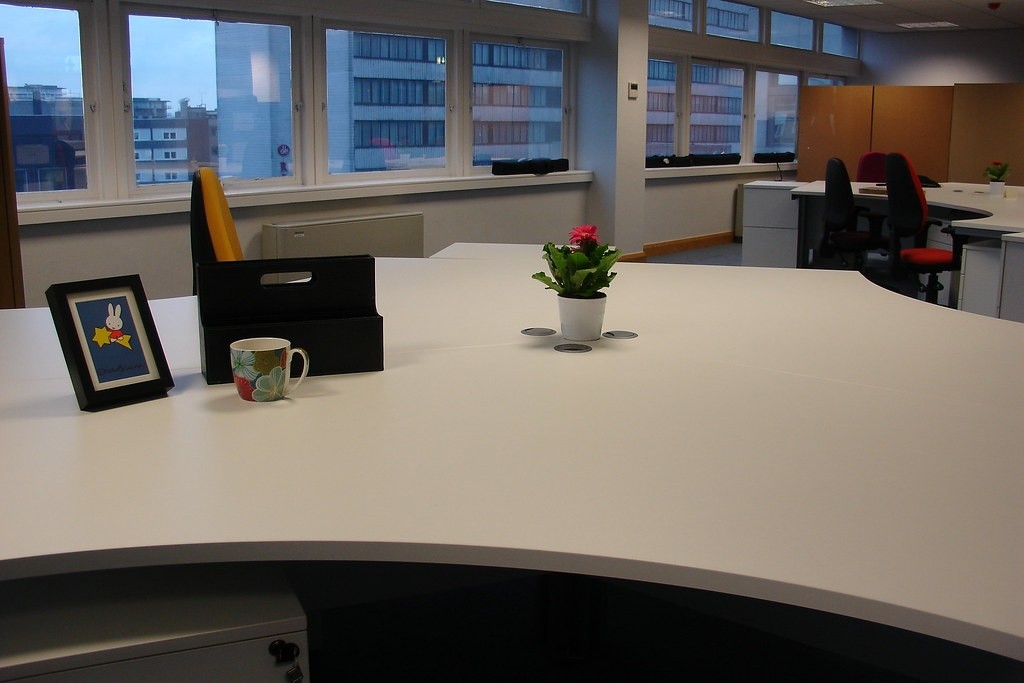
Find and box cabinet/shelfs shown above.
[958,239,1001,317]
[797,85,874,182]
[871,82,953,183]
[948,81,1024,186]
[0,565,311,683]
[743,181,808,268]
[917,216,956,306]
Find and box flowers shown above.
[984,160,1012,181]
[532,224,617,294]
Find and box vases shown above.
[989,182,1006,199]
[558,292,607,341]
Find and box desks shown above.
[0,243,1024,663]
[789,180,1024,323]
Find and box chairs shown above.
[819,159,889,286]
[886,153,953,305]
[856,150,890,183]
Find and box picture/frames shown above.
[45,274,174,410]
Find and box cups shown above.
[230,337,309,402]
